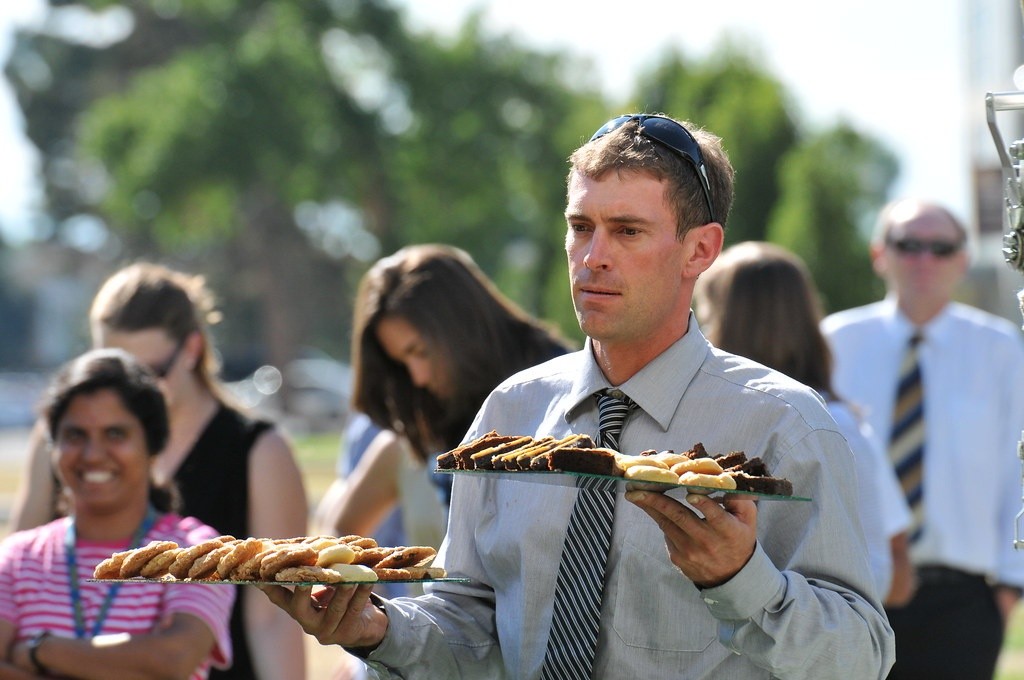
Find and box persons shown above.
[304,240,579,599]
[0,345,236,679]
[694,244,916,611]
[807,197,1024,680]
[260,109,900,680]
[10,262,311,680]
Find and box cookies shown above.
[92,536,450,582]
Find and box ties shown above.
[540,387,639,680]
[888,335,924,544]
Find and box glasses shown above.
[886,236,965,257]
[589,114,716,222]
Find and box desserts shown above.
[439,433,792,496]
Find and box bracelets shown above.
[27,631,51,676]
